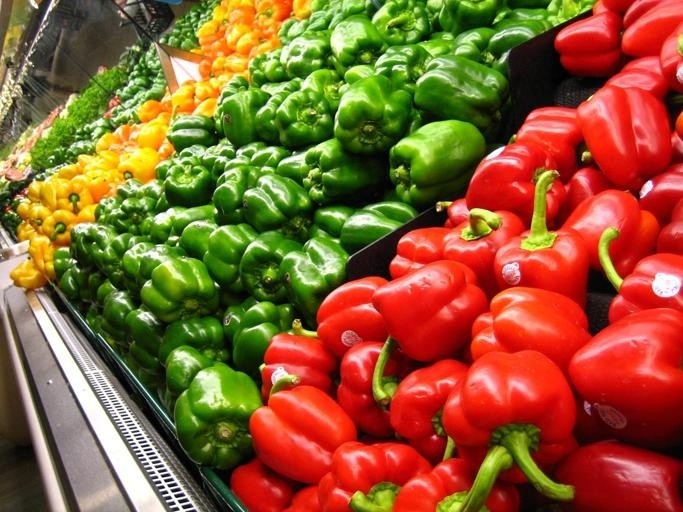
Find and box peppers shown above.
[230,1,683,512]
[0,1,318,291]
[51,0,596,472]
